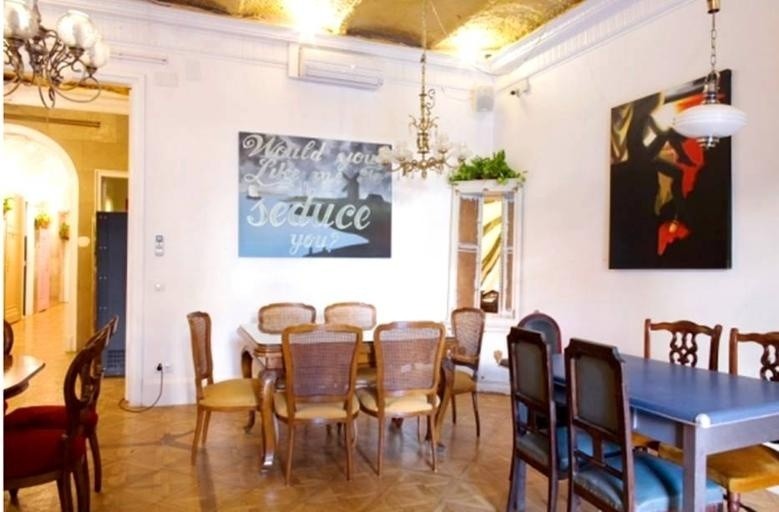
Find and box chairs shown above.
[507,315,779,511]
[4,321,13,355]
[5,314,119,499]
[5,323,111,511]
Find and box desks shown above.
[5,355,45,398]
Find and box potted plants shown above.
[449,150,527,193]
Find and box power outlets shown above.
[155,364,169,375]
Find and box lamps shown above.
[671,0,746,149]
[4,0,110,126]
[375,0,466,178]
